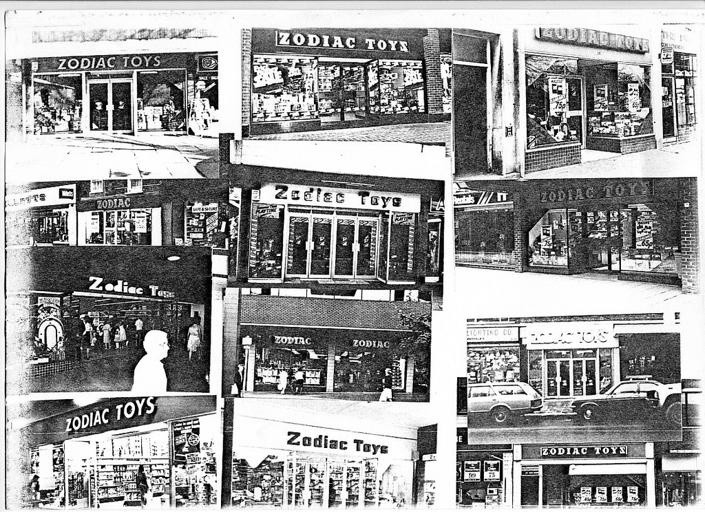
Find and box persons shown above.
[187,319,201,361]
[28,475,40,491]
[128,329,170,393]
[382,368,392,401]
[136,465,149,504]
[329,478,336,507]
[278,368,307,394]
[81,315,143,350]
[234,363,244,397]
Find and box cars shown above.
[466,379,545,425]
[646,381,682,423]
[571,376,663,422]
